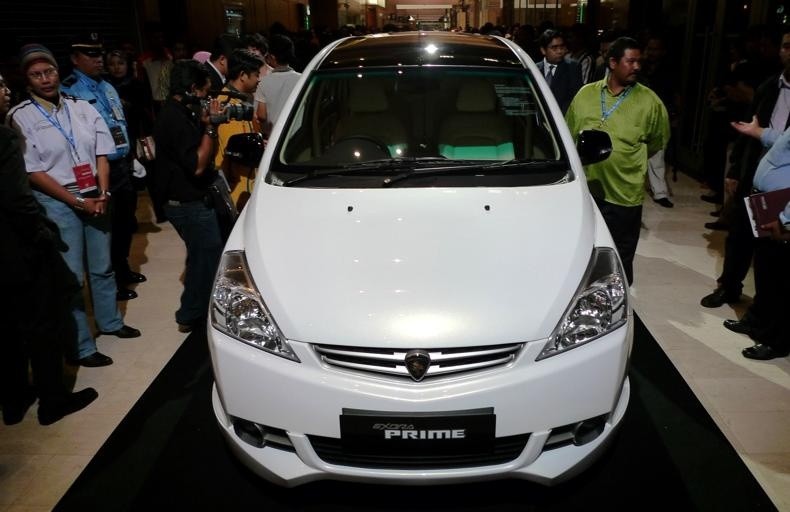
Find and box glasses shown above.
[26,67,57,79]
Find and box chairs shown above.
[331,84,517,159]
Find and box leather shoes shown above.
[701,195,728,231]
[98,325,141,337]
[79,352,112,367]
[723,319,758,340]
[116,270,147,301]
[2,383,98,426]
[742,343,790,360]
[701,288,740,307]
[653,197,674,208]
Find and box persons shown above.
[304,20,503,69]
[1,23,305,428]
[503,12,789,362]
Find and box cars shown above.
[207,30,633,498]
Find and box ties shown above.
[545,64,555,87]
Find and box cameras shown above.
[184,91,254,124]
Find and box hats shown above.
[20,43,58,75]
[67,30,108,57]
